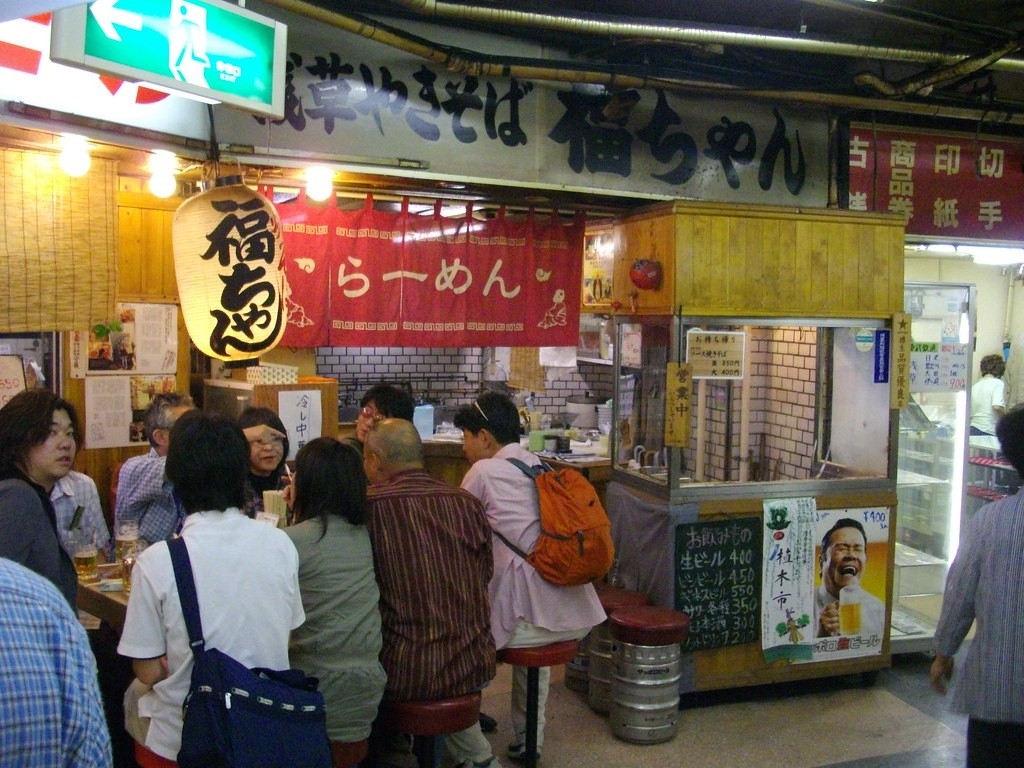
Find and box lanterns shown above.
[170,176,289,369]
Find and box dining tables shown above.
[72,569,129,632]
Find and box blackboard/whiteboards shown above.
[0,354,27,412]
[673,515,764,653]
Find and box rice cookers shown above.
[564,392,612,433]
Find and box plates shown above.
[596,403,614,429]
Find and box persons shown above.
[437,393,608,768]
[361,417,495,768]
[240,407,288,492]
[0,479,112,768]
[113,392,287,544]
[971,354,1005,435]
[116,408,305,768]
[281,436,386,746]
[927,406,1024,768]
[0,390,139,768]
[814,519,882,637]
[339,385,412,459]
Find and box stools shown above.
[566,581,691,745]
[378,690,482,767]
[495,639,577,767]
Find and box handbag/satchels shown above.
[176,647,332,768]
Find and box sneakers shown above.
[508,740,542,760]
[462,756,503,768]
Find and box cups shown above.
[527,430,560,454]
[833,583,863,637]
[71,529,99,580]
[121,542,143,596]
[114,516,141,564]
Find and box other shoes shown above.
[479,712,497,731]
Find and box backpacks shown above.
[492,457,615,586]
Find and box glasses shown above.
[472,399,489,421]
[360,407,383,423]
